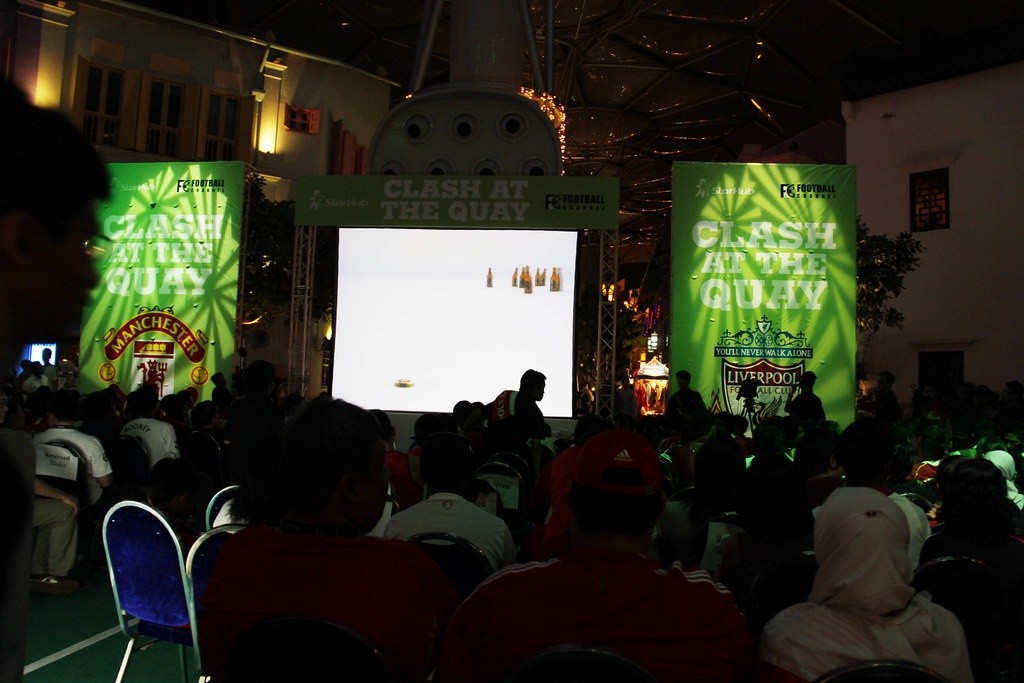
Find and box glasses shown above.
[1000,389,1016,395]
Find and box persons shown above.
[0,75,1023,682]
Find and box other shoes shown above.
[27,575,77,593]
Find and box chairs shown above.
[34,428,1024,683]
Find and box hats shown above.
[571,427,661,496]
